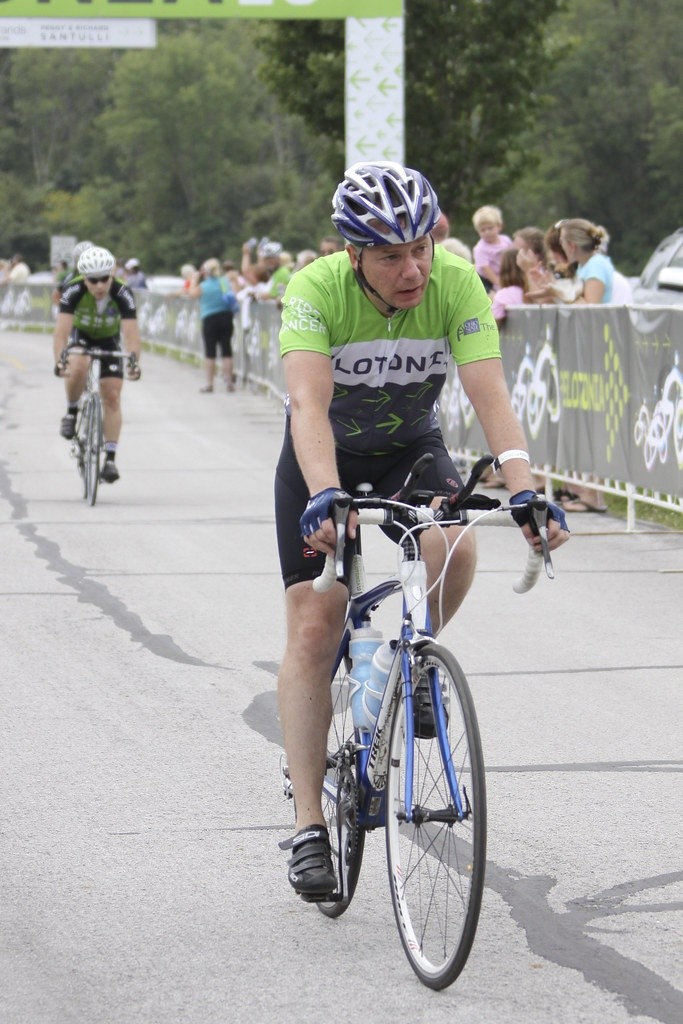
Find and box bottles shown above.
[361,639,399,727]
[347,616,385,728]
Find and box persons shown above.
[1,253,149,292]
[180,237,342,398]
[274,162,572,892]
[53,245,141,484]
[430,204,630,333]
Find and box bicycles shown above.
[276,491,559,995]
[57,347,137,509]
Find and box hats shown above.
[124,259,139,270]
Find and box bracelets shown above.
[491,449,531,473]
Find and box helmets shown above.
[331,162,441,247]
[74,242,94,259]
[77,247,115,275]
[260,243,281,258]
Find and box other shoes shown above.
[228,384,235,393]
[199,386,211,392]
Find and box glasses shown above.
[84,275,109,284]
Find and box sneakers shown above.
[413,674,449,737]
[100,462,119,483]
[61,414,76,438]
[277,824,337,893]
